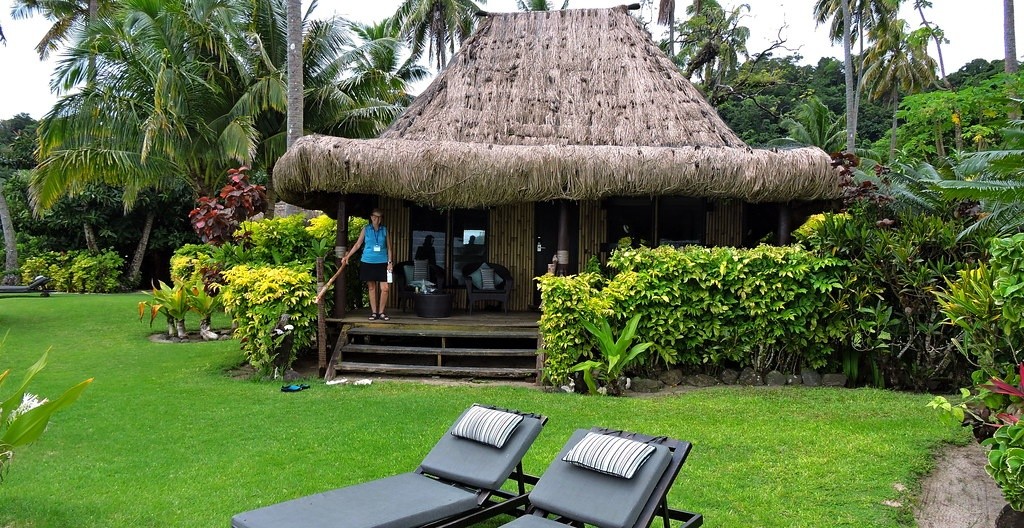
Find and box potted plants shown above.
[567,307,669,396]
[208,259,335,374]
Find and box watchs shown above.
[388,262,391,264]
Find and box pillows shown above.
[404,259,437,287]
[478,267,496,290]
[450,404,525,450]
[35,276,43,280]
[468,262,504,289]
[560,429,657,479]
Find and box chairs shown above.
[0,276,50,297]
[228,402,548,528]
[498,425,703,528]
[390,258,447,311]
[463,262,514,315]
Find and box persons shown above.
[341,209,393,321]
[415,235,436,266]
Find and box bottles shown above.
[386,261,393,284]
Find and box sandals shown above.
[368,313,378,320]
[378,313,390,321]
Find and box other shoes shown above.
[281,385,301,392]
[298,383,310,389]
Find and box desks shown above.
[413,291,453,319]
